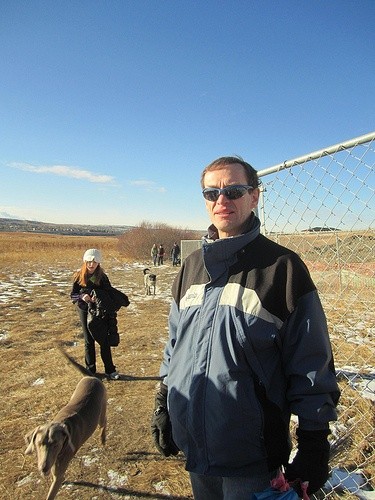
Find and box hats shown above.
[84,249,101,264]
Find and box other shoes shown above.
[106,371,121,380]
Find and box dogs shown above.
[143,268,157,296]
[23,341,106,500]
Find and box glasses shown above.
[202,185,254,201]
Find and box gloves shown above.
[285,428,331,495]
[151,386,178,458]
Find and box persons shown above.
[150,244,158,266]
[151,157,340,499]
[70,250,120,381]
[157,243,164,266]
[170,242,180,265]
